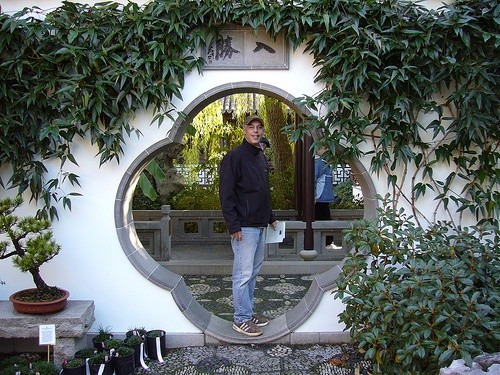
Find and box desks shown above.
[0,300,95,370]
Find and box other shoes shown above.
[326,244,342,249]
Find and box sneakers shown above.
[251,314,269,327]
[233,320,263,337]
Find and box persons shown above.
[259,138,270,153]
[314,144,342,250]
[219,114,279,337]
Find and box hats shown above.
[245,115,264,125]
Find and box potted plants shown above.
[0,196,70,314]
[62,323,167,375]
